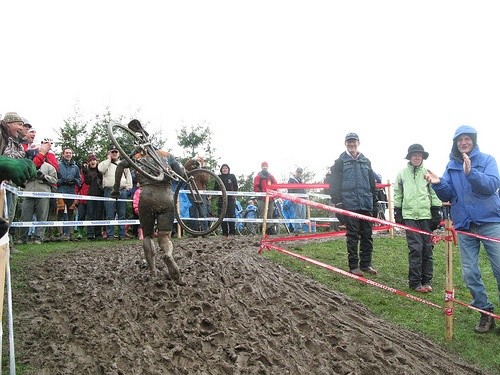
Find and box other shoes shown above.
[42,233,126,242]
[14,238,27,245]
[361,266,377,275]
[349,268,364,276]
[474,311,496,333]
[32,238,42,244]
[414,284,433,293]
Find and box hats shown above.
[88,154,96,162]
[345,132,359,141]
[108,144,118,151]
[20,117,32,128]
[4,111,24,123]
[296,168,303,174]
[261,161,268,166]
[404,144,429,160]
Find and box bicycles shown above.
[236,197,291,237]
[108,119,228,236]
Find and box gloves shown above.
[430,213,442,232]
[0,157,36,183]
[373,206,380,212]
[335,203,346,225]
[60,176,74,185]
[394,212,404,224]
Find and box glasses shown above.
[110,151,118,153]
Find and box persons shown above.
[287,167,307,234]
[393,144,443,293]
[0,112,295,254]
[423,125,500,332]
[329,133,380,276]
[214,164,238,237]
[110,149,188,280]
[254,162,279,235]
[323,166,388,231]
